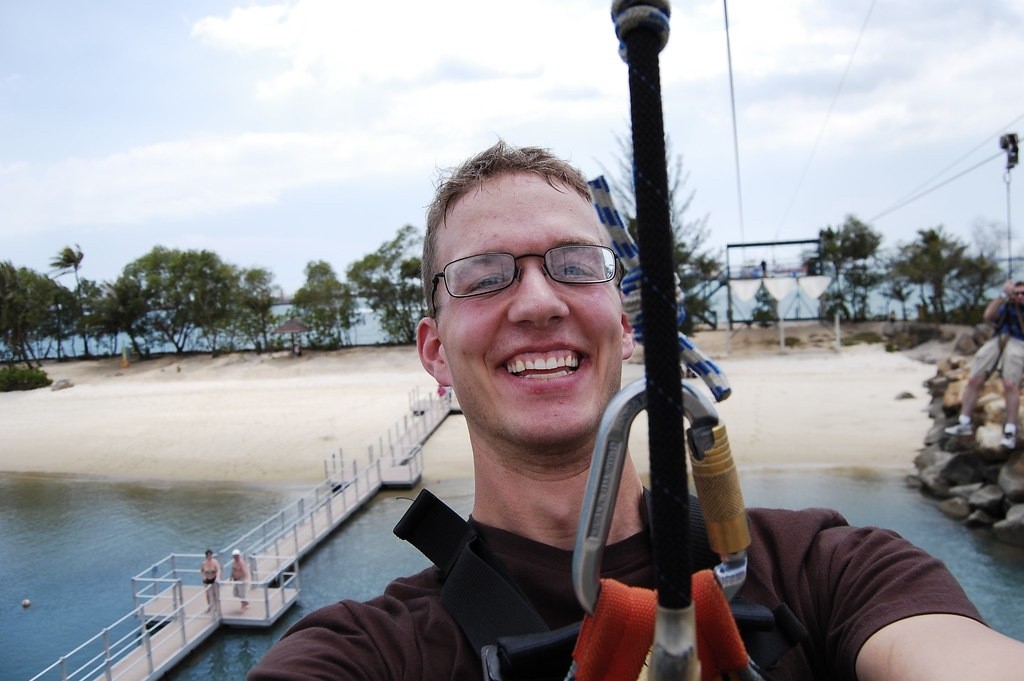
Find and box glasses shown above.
[432,245,624,316]
[1015,291,1024,296]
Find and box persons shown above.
[200,550,221,613]
[942,279,1024,449]
[243,144,1023,681]
[229,549,251,609]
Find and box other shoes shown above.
[945,422,973,437]
[1001,431,1017,449]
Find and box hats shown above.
[232,550,240,556]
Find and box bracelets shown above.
[999,291,1010,302]
